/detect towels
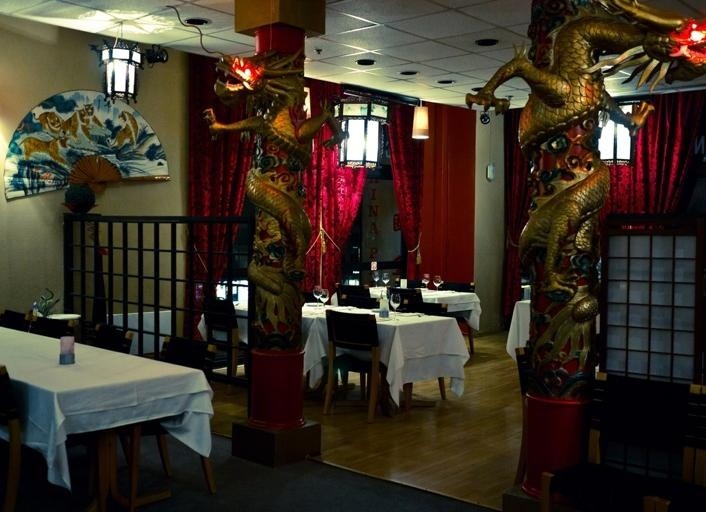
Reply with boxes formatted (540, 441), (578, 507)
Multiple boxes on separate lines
(335, 306), (357, 311)
(396, 312), (424, 317)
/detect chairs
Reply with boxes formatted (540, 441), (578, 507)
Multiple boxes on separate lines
(111, 337), (215, 512)
(0, 366), (95, 512)
(439, 281), (475, 355)
(387, 289), (447, 398)
(81, 323), (129, 353)
(0, 309), (25, 331)
(387, 288), (424, 313)
(336, 286), (372, 398)
(323, 311), (410, 421)
(203, 298), (246, 382)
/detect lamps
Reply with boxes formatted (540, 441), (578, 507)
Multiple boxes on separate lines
(413, 99), (430, 139)
(92, 36), (141, 102)
(599, 103), (639, 166)
(333, 89), (388, 168)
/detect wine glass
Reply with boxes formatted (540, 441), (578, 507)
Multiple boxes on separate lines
(381, 273), (390, 288)
(373, 270), (379, 289)
(433, 275), (442, 291)
(390, 294), (401, 320)
(421, 273), (431, 292)
(313, 285), (322, 307)
(321, 289), (330, 308)
